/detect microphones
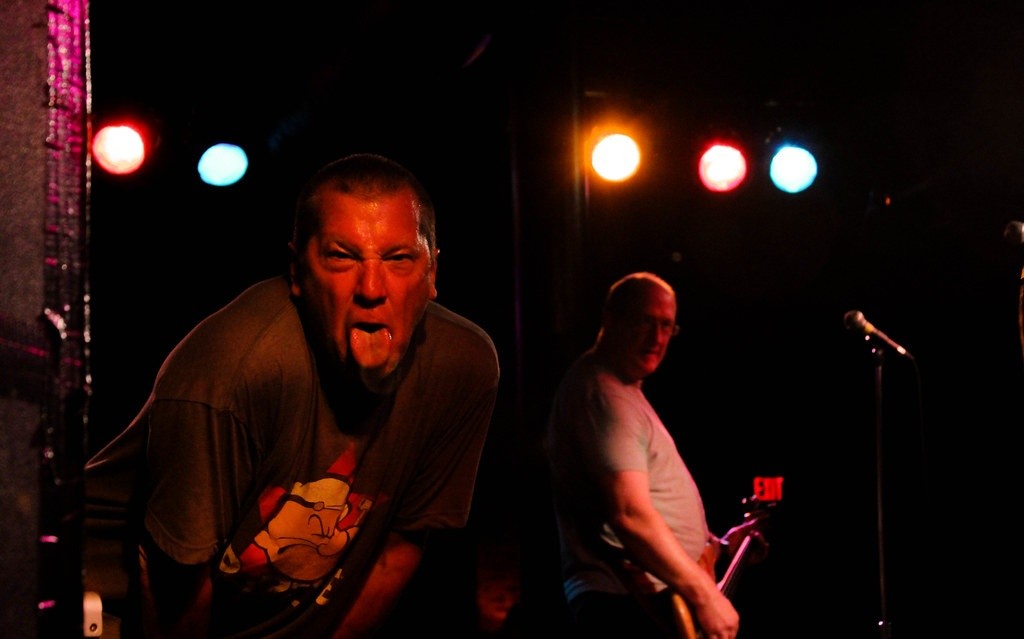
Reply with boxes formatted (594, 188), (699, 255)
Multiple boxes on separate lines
(845, 310), (914, 360)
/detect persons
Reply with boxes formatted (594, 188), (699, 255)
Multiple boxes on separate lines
(60, 153), (501, 639)
(542, 273), (772, 639)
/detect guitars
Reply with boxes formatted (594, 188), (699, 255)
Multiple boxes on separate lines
(666, 478), (783, 639)
